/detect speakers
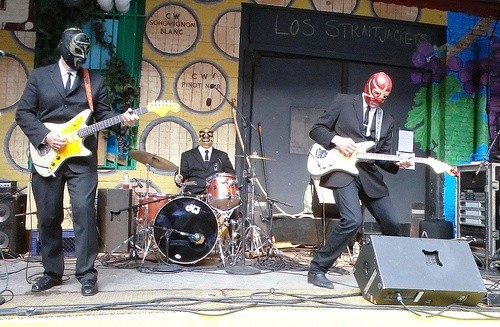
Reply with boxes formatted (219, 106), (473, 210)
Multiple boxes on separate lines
(353, 234), (486, 307)
(312, 178), (365, 218)
(0, 192), (27, 257)
(98, 189), (132, 254)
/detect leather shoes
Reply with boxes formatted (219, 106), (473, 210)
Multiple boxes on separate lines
(31, 275), (62, 292)
(81, 280), (98, 295)
(308, 270), (335, 289)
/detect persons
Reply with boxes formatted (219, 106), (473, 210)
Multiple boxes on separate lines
(174, 127), (242, 263)
(308, 72), (410, 289)
(15, 27), (139, 296)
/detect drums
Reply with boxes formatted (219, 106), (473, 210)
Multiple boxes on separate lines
(206, 173), (241, 210)
(152, 197), (218, 264)
(137, 192), (171, 225)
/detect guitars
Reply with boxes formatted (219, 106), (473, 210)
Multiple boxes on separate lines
(30, 99), (181, 177)
(307, 141), (457, 177)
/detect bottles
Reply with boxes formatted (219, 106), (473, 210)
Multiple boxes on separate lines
(352, 242), (359, 260)
(122, 171), (130, 189)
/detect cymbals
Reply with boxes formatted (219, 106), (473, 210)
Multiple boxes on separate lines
(231, 154), (272, 161)
(129, 150), (179, 173)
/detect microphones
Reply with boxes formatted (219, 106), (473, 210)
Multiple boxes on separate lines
(206, 77), (214, 106)
(175, 230), (201, 241)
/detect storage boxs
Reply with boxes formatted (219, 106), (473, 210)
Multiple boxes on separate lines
(32, 229), (76, 257)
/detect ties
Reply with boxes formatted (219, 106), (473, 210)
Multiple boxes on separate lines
(205, 150), (209, 167)
(65, 72), (72, 95)
(362, 105), (370, 136)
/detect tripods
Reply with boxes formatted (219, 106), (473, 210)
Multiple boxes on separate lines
(233, 158), (283, 264)
(96, 163), (179, 270)
